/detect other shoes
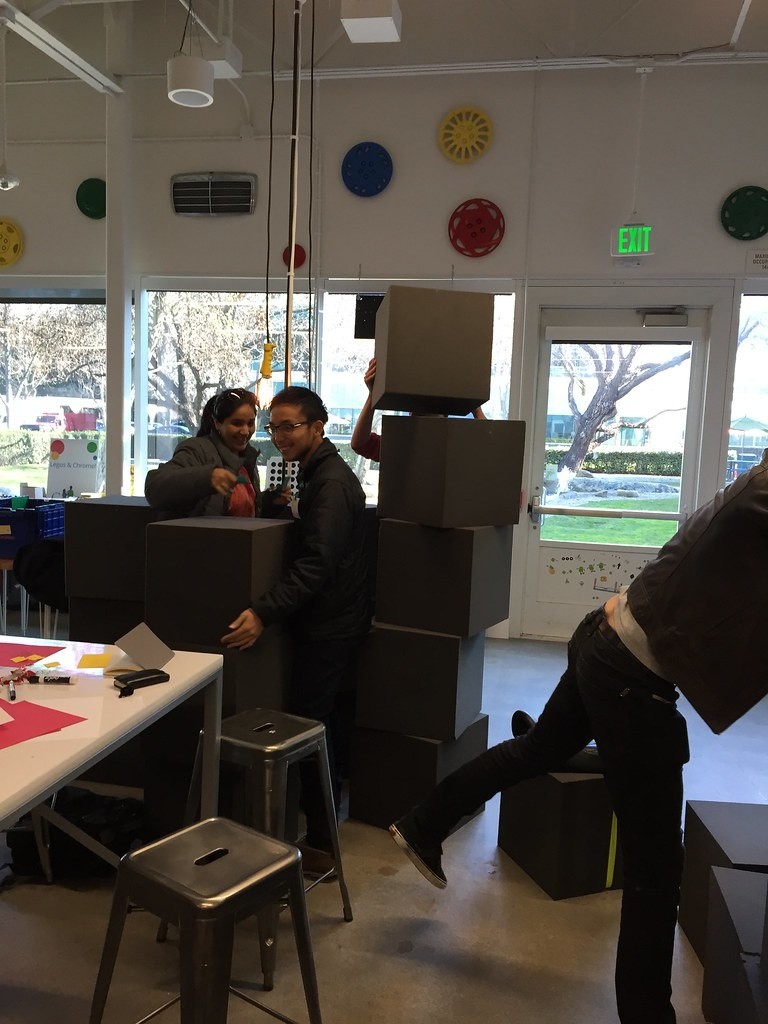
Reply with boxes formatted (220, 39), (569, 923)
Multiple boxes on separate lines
(290, 834), (339, 878)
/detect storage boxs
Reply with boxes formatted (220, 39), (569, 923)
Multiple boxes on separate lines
(373, 518), (513, 637)
(68, 598), (144, 645)
(353, 621), (486, 742)
(20, 487), (43, 499)
(678, 798), (768, 966)
(362, 503), (386, 614)
(739, 952), (768, 1024)
(0, 496), (64, 560)
(56, 738), (201, 842)
(143, 515), (296, 647)
(370, 285), (495, 417)
(346, 712), (489, 839)
(702, 865), (768, 1024)
(63, 494), (169, 602)
(141, 639), (299, 768)
(498, 767), (625, 901)
(378, 413), (527, 529)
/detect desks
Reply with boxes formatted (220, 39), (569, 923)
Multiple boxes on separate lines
(0, 633), (225, 884)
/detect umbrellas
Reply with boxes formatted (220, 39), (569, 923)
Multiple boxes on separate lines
(730, 416), (768, 459)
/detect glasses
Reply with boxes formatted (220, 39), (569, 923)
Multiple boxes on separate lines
(228, 388), (246, 401)
(264, 421), (311, 436)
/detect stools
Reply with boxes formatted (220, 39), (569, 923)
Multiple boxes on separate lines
(154, 705), (354, 993)
(87, 814), (325, 1024)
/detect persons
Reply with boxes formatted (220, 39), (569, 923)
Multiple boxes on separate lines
(144, 388), (292, 519)
(387, 453), (768, 1024)
(220, 384), (374, 884)
(351, 359), (488, 461)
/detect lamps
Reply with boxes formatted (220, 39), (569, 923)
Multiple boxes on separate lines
(166, 55), (216, 109)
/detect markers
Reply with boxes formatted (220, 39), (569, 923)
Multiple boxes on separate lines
(27, 676), (71, 685)
(9, 680), (16, 701)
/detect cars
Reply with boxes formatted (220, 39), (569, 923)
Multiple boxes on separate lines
(148, 425), (190, 434)
(20, 413), (65, 431)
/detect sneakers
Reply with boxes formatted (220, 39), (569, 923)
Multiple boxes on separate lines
(387, 804), (446, 889)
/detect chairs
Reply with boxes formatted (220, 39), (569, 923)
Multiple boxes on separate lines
(13, 536), (70, 638)
